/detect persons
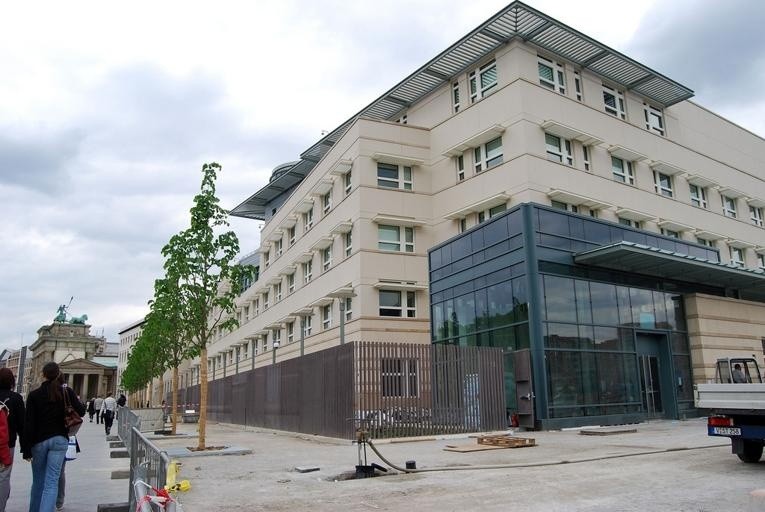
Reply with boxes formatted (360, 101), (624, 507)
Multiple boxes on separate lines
(114, 393), (127, 421)
(86, 397), (95, 422)
(76, 394), (84, 406)
(730, 363), (748, 383)
(52, 369), (66, 511)
(0, 366), (27, 511)
(20, 360), (85, 512)
(98, 392), (117, 435)
(92, 395), (104, 424)
(58, 304), (68, 314)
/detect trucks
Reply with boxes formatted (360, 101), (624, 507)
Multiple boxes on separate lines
(693, 383), (765, 463)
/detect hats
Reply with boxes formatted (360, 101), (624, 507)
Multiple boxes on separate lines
(42, 361), (59, 377)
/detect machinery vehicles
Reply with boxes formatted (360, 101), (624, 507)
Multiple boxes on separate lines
(714, 356), (763, 382)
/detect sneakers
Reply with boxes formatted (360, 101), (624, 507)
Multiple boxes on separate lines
(107, 427), (111, 434)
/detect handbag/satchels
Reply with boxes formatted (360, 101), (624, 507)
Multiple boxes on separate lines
(61, 385), (84, 437)
(64, 435), (77, 461)
